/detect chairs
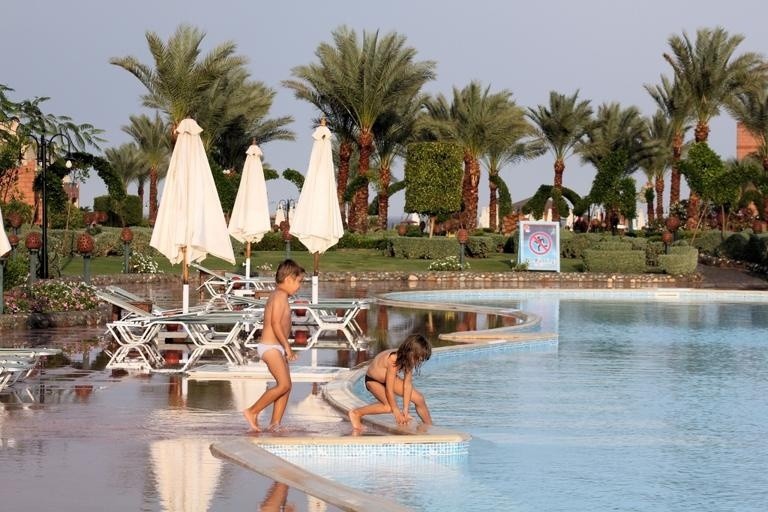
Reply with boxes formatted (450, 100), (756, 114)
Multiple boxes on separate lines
(1, 344), (64, 404)
(95, 262), (370, 376)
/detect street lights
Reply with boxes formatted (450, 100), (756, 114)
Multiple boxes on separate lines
(279, 198), (295, 259)
(18, 133), (73, 279)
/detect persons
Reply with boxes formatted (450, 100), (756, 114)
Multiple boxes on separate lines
(346, 331), (434, 432)
(257, 479), (294, 511)
(241, 258), (308, 432)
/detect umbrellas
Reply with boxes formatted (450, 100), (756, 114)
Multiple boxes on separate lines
(290, 343), (345, 512)
(287, 119), (347, 343)
(0, 208), (12, 261)
(229, 379), (269, 416)
(148, 115), (236, 360)
(227, 136), (272, 333)
(152, 362), (226, 511)
